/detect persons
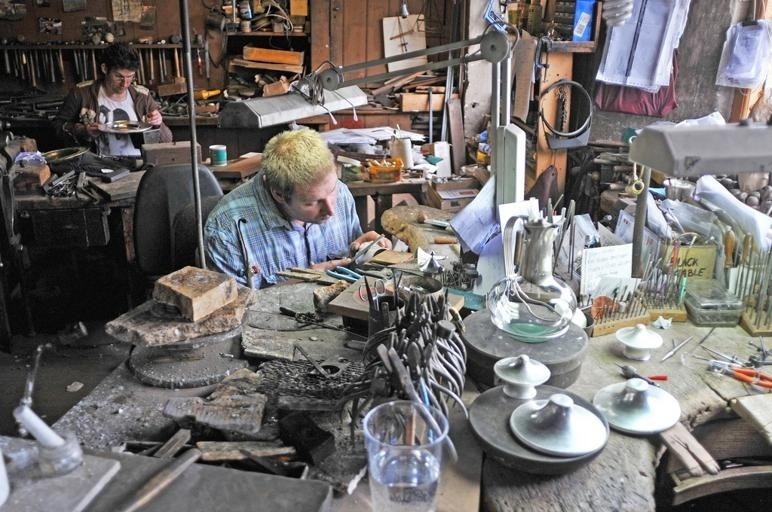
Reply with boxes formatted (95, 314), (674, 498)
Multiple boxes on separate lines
(194, 129), (393, 292)
(52, 42), (173, 160)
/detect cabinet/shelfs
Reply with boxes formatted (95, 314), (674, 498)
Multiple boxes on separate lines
(326, 107), (415, 131)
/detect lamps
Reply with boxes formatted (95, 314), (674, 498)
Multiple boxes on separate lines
(626, 119), (771, 179)
(216, 20), (527, 223)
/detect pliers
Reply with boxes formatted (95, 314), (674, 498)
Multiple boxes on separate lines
(326, 265), (362, 281)
(708, 359), (772, 388)
(376, 290), (468, 464)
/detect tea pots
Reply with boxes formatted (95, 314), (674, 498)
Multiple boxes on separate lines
(487, 213), (576, 344)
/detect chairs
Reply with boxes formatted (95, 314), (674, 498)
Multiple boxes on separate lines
(133, 163), (223, 276)
(175, 195), (224, 268)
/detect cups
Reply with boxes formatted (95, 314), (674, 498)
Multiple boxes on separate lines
(209, 144), (228, 167)
(364, 400), (450, 512)
